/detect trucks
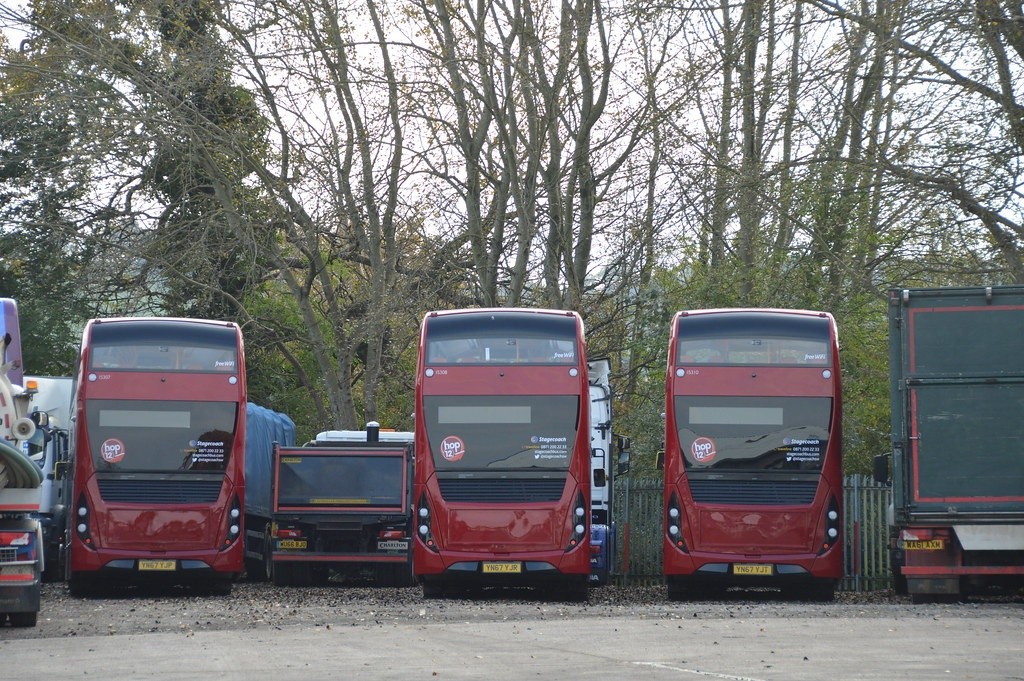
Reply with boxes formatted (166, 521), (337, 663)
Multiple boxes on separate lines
(1, 298), (73, 628)
(873, 284), (1024, 606)
(265, 421), (414, 588)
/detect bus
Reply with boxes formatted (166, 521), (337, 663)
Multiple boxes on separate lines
(657, 308), (844, 603)
(587, 352), (632, 589)
(66, 316), (247, 599)
(416, 307), (594, 605)
